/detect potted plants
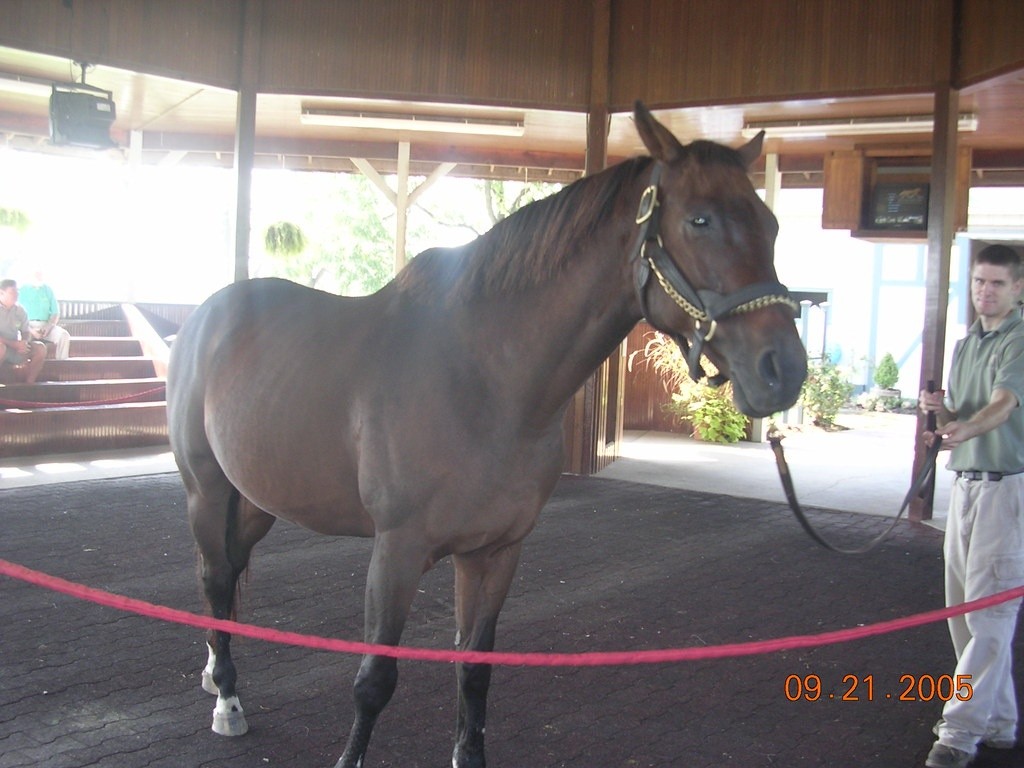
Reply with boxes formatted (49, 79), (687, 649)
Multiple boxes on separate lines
(870, 353), (900, 408)
(628, 329), (750, 445)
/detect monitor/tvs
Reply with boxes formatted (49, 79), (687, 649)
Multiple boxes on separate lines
(861, 157), (927, 231)
(48, 91), (116, 150)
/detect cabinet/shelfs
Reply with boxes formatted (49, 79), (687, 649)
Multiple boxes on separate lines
(822, 143), (974, 243)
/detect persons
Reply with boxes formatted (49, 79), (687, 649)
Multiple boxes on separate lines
(17, 265), (70, 360)
(919, 244), (1024, 768)
(0, 279), (47, 383)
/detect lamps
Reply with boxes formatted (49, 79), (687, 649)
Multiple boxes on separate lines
(742, 113), (979, 132)
(301, 107), (525, 137)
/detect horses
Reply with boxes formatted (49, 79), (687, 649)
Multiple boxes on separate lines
(165, 92), (810, 767)
